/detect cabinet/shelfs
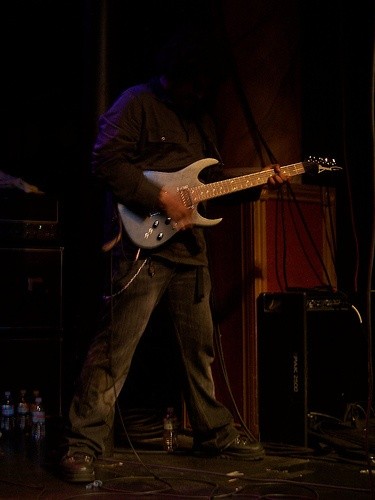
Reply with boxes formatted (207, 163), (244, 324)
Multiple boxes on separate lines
(1, 244), (65, 419)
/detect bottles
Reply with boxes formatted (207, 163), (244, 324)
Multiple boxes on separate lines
(30, 398), (45, 441)
(163, 408), (178, 453)
(0, 390), (40, 432)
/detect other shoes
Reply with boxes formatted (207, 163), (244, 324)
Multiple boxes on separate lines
(61, 452), (96, 482)
(192, 434), (265, 461)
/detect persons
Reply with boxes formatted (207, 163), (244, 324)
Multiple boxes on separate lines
(58, 37), (289, 483)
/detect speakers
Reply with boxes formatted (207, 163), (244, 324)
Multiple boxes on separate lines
(256, 287), (369, 455)
(0, 246), (64, 333)
(0, 334), (64, 420)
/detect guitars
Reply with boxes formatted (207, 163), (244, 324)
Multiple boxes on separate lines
(115, 156), (344, 251)
